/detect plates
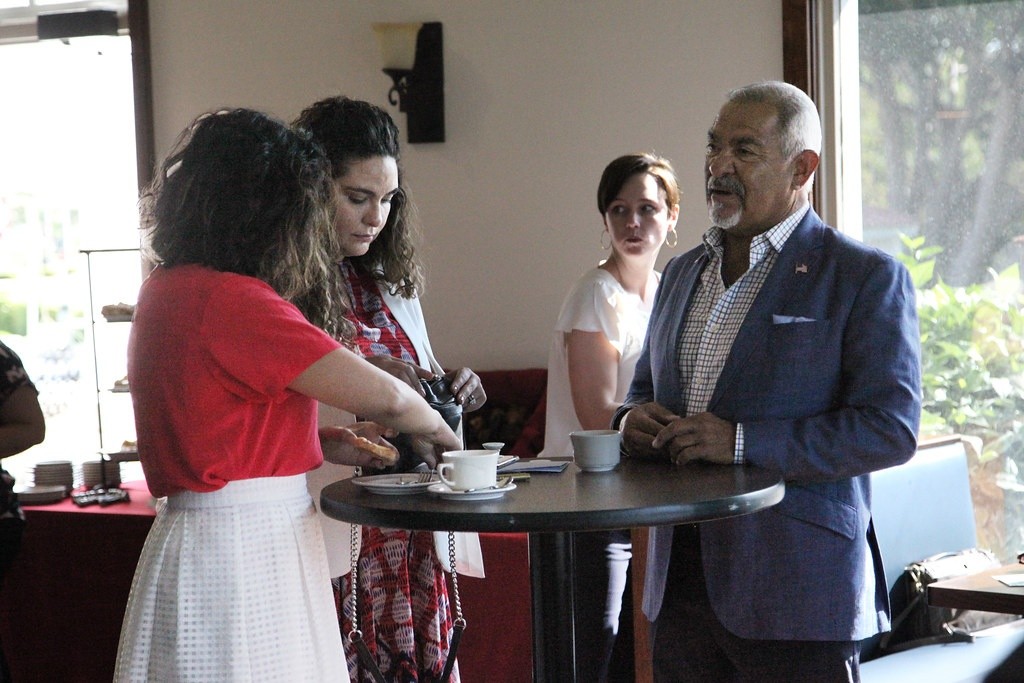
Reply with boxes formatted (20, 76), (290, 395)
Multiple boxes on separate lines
(427, 482), (517, 500)
(351, 473), (440, 494)
(16, 485), (65, 503)
(81, 461), (121, 489)
(30, 461), (74, 495)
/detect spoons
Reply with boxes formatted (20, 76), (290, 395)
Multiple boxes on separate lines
(464, 475), (513, 493)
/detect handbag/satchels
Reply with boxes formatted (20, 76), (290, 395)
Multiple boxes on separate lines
(359, 370), (463, 475)
(878, 548), (1021, 658)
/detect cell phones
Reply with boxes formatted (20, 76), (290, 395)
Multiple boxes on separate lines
(497, 455), (519, 469)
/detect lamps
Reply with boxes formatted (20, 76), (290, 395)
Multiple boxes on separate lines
(371, 21), (444, 144)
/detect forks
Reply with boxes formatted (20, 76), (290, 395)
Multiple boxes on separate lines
(418, 470), (433, 482)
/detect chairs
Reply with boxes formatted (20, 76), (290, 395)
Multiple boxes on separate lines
(860, 436), (1024, 683)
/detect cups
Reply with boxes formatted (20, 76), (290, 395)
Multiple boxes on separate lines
(438, 450), (500, 492)
(569, 430), (621, 472)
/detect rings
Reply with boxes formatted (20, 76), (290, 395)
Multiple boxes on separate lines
(468, 395), (476, 405)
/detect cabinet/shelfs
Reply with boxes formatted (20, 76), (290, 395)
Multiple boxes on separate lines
(80, 248), (140, 491)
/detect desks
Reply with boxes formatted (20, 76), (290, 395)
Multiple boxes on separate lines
(319, 456), (787, 683)
(10, 479), (157, 682)
(927, 560), (1024, 615)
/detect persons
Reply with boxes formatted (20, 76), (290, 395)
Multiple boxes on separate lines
(112, 104), (466, 683)
(527, 151), (682, 682)
(0, 343), (47, 681)
(616, 77), (920, 682)
(285, 93), (491, 683)
